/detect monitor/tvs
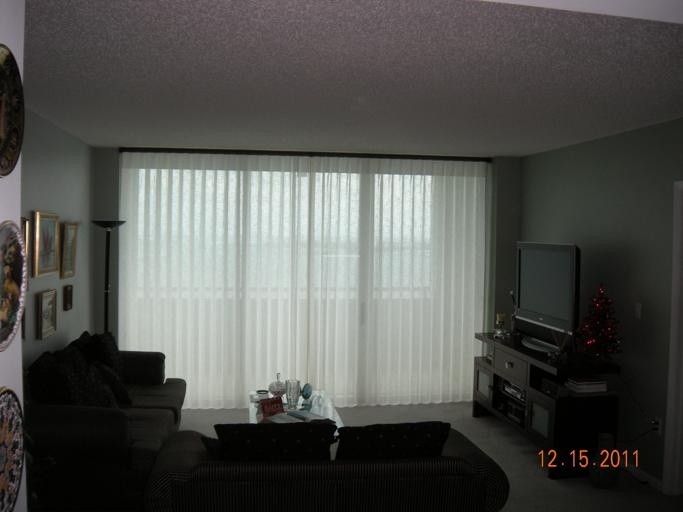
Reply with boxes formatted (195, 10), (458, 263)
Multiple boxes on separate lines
(514, 241), (580, 352)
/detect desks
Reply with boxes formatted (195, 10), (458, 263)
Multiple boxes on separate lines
(246, 391), (345, 436)
(472, 323), (621, 454)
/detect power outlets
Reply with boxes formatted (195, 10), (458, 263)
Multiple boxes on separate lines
(650, 418), (664, 436)
(633, 302), (644, 321)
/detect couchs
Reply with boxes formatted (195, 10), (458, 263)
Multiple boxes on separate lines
(149, 429), (510, 512)
(19, 349), (186, 478)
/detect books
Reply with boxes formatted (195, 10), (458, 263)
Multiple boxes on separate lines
(264, 410), (326, 423)
(564, 377), (607, 394)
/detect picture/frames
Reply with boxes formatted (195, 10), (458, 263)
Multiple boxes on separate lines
(38, 288), (58, 342)
(31, 208), (60, 280)
(64, 284), (73, 311)
(61, 221), (78, 278)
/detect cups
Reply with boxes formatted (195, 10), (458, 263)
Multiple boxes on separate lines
(256, 390), (269, 401)
(286, 380), (302, 410)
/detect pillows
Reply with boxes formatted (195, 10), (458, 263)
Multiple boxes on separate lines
(75, 328), (130, 388)
(35, 347), (117, 414)
(338, 419), (450, 459)
(214, 419), (335, 465)
